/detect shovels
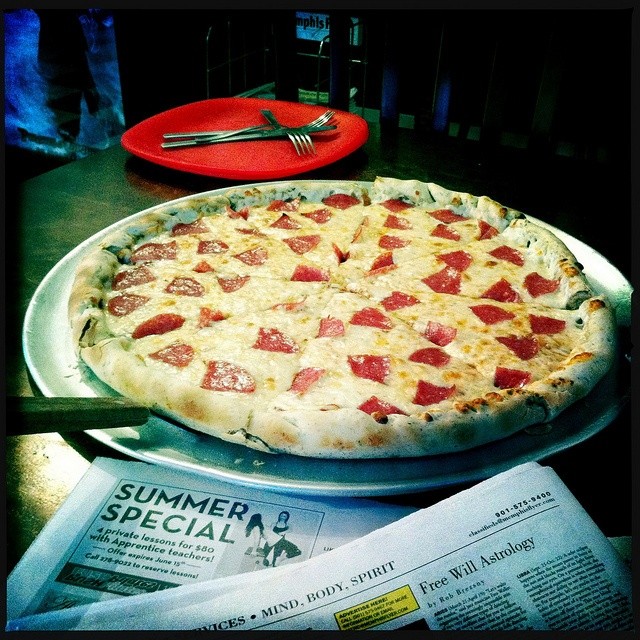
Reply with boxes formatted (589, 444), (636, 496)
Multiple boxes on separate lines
(5, 395), (209, 438)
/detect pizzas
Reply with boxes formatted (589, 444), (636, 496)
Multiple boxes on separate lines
(66, 175), (615, 462)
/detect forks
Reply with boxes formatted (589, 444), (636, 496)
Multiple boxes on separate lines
(163, 112), (342, 151)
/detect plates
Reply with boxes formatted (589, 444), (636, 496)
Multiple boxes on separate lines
(22, 180), (633, 496)
(119, 94), (371, 179)
